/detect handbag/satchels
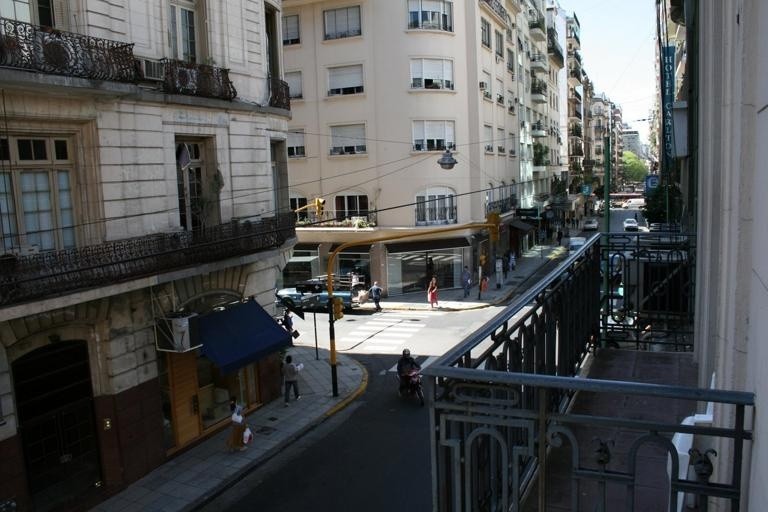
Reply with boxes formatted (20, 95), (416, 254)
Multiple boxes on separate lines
(231, 411), (243, 424)
(242, 427), (254, 445)
(283, 320), (289, 327)
(292, 329), (300, 339)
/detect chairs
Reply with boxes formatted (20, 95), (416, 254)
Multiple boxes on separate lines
(32, 31), (84, 76)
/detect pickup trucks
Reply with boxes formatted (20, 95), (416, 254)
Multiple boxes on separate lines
(274, 273), (366, 311)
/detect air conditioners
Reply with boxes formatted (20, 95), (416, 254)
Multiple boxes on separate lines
(172, 66), (198, 91)
(521, 121), (529, 128)
(133, 58), (166, 83)
(479, 80), (487, 91)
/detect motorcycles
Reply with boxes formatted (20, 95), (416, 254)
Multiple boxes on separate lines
(401, 367), (424, 405)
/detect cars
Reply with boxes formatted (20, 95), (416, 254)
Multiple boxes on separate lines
(583, 218), (599, 231)
(568, 236), (586, 257)
(624, 217), (638, 231)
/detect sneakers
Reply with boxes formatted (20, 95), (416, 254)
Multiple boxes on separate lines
(296, 395), (302, 401)
(239, 446), (248, 452)
(284, 402), (290, 407)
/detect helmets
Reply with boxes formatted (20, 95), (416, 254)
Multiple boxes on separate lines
(403, 348), (410, 358)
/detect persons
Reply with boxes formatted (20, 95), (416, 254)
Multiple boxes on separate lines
(482, 209), (581, 296)
(280, 356), (302, 407)
(461, 265), (470, 297)
(367, 281), (383, 312)
(396, 348), (420, 396)
(283, 308), (294, 347)
(228, 405), (248, 452)
(427, 274), (438, 308)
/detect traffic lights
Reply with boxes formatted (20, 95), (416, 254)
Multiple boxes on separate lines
(315, 198), (325, 217)
(334, 296), (344, 320)
(480, 254), (486, 268)
